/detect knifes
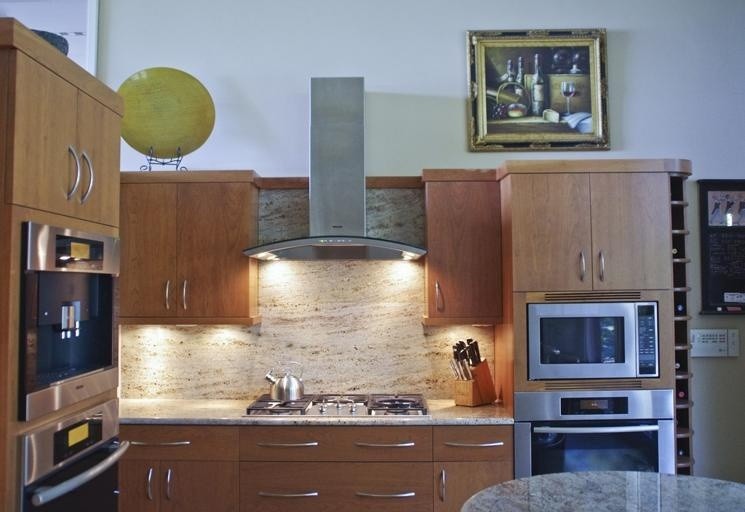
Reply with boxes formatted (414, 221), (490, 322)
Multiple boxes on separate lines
(447, 336), (498, 408)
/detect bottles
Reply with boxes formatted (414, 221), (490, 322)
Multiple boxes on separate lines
(505, 59), (516, 93)
(515, 56), (526, 96)
(530, 54), (550, 116)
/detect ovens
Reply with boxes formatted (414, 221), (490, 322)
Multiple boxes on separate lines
(20, 228), (123, 420)
(16, 397), (126, 511)
(515, 394), (675, 475)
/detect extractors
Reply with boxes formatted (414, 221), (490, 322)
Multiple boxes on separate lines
(242, 75), (427, 265)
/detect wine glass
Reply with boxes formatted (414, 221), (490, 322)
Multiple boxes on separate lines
(561, 81), (577, 117)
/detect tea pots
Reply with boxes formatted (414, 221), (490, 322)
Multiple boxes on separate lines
(265, 359), (307, 401)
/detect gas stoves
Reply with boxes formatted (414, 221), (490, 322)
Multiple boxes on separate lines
(247, 390), (429, 417)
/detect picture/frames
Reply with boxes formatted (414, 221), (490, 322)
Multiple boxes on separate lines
(462, 26), (613, 153)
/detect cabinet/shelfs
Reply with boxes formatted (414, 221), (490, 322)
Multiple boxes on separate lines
(434, 425), (515, 511)
(0, 15), (124, 226)
(120, 424), (240, 512)
(490, 156), (692, 293)
(414, 163), (504, 326)
(241, 424), (433, 512)
(117, 169), (261, 326)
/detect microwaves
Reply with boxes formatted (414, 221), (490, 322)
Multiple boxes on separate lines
(528, 302), (659, 380)
